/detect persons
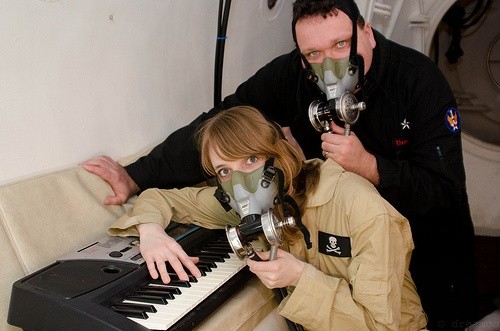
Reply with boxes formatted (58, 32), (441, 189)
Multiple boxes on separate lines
(80, 0), (480, 331)
(124, 105), (430, 331)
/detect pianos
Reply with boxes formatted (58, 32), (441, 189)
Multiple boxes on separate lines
(7, 217), (259, 330)
